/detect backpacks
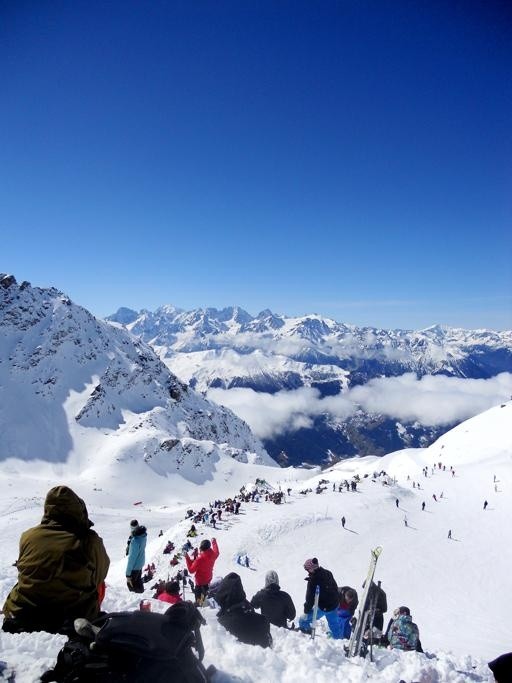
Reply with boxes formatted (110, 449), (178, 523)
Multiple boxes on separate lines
(40, 599), (216, 683)
(216, 603), (271, 648)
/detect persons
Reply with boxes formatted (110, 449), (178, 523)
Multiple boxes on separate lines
(251, 569), (298, 628)
(335, 584), (360, 639)
(362, 578), (388, 644)
(448, 530), (451, 538)
(286, 469), (388, 496)
(341, 517), (345, 527)
(395, 462), (454, 526)
(300, 556), (344, 639)
(383, 604), (424, 653)
(210, 478), (283, 514)
(483, 500), (488, 509)
(146, 508), (223, 603)
(213, 570), (276, 651)
(238, 555), (249, 567)
(124, 518), (150, 592)
(2, 483), (111, 636)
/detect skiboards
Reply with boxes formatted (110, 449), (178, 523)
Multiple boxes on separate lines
(310, 586), (320, 640)
(343, 546), (382, 660)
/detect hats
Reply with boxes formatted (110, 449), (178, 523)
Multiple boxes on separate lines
(131, 520), (139, 529)
(304, 558), (318, 571)
(265, 570), (279, 586)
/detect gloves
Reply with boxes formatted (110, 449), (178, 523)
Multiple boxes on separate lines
(127, 577), (133, 588)
